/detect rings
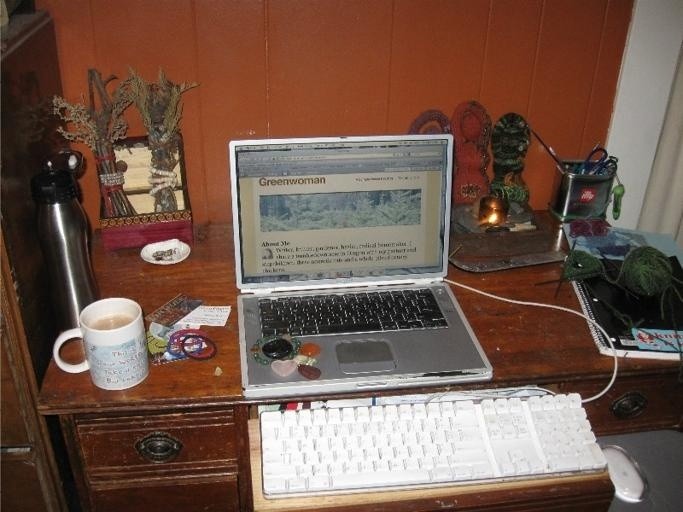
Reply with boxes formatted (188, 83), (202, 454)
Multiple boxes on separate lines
(613, 184), (625, 220)
(573, 141), (618, 175)
(532, 129), (567, 172)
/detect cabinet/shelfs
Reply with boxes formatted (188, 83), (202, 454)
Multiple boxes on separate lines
(31, 168), (98, 329)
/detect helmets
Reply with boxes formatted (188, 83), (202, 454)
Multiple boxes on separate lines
(141, 238), (190, 265)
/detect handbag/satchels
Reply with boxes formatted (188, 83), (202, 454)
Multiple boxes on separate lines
(250, 331), (301, 366)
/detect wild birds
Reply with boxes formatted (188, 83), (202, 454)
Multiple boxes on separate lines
(228, 134), (494, 399)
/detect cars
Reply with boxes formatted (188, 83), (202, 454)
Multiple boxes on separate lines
(53, 297), (148, 391)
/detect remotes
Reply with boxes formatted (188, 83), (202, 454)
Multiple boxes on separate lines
(602, 444), (650, 505)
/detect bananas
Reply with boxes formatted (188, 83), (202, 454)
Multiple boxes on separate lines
(258, 392), (608, 499)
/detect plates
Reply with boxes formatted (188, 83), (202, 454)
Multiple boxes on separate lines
(0, 12), (61, 512)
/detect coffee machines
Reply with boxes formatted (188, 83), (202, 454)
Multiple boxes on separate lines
(584, 147), (617, 175)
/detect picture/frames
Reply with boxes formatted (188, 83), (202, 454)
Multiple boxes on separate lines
(35, 210), (683, 512)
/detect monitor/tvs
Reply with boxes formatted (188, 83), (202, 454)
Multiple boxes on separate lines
(563, 253), (683, 360)
